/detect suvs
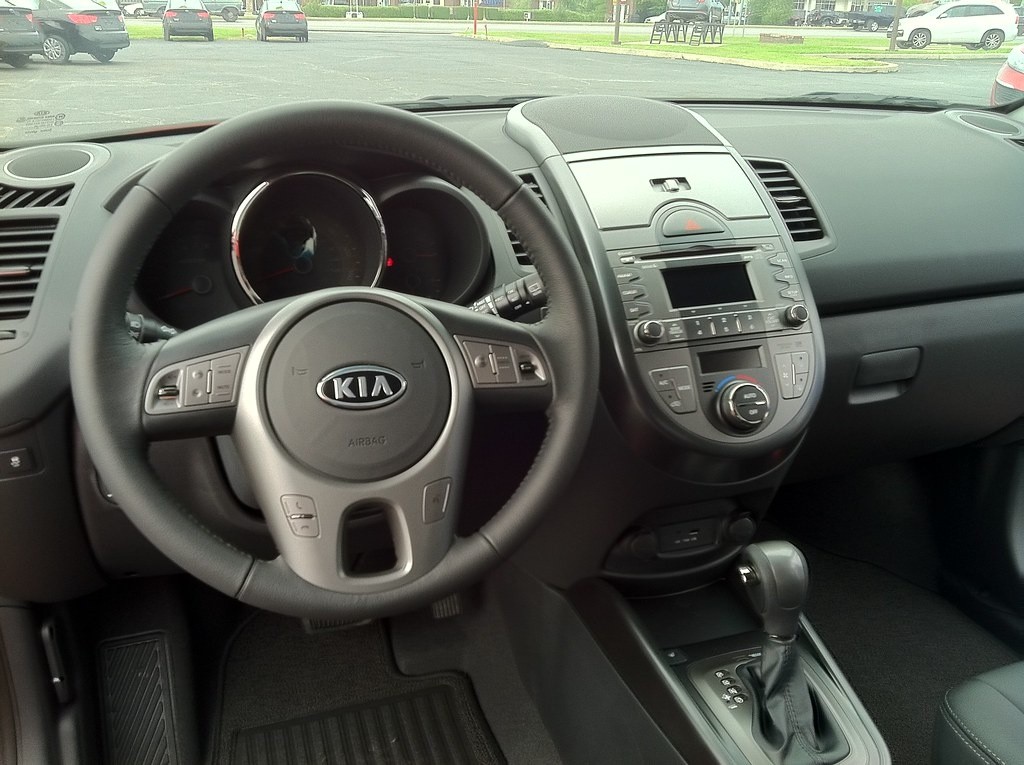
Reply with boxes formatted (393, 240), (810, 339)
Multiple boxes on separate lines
(123, 0), (246, 23)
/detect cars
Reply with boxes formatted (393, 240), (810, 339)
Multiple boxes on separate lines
(805, 0), (1024, 50)
(162, 0), (213, 41)
(642, 0), (748, 25)
(255, 0), (309, 43)
(29, 0), (132, 65)
(0, 6), (45, 69)
